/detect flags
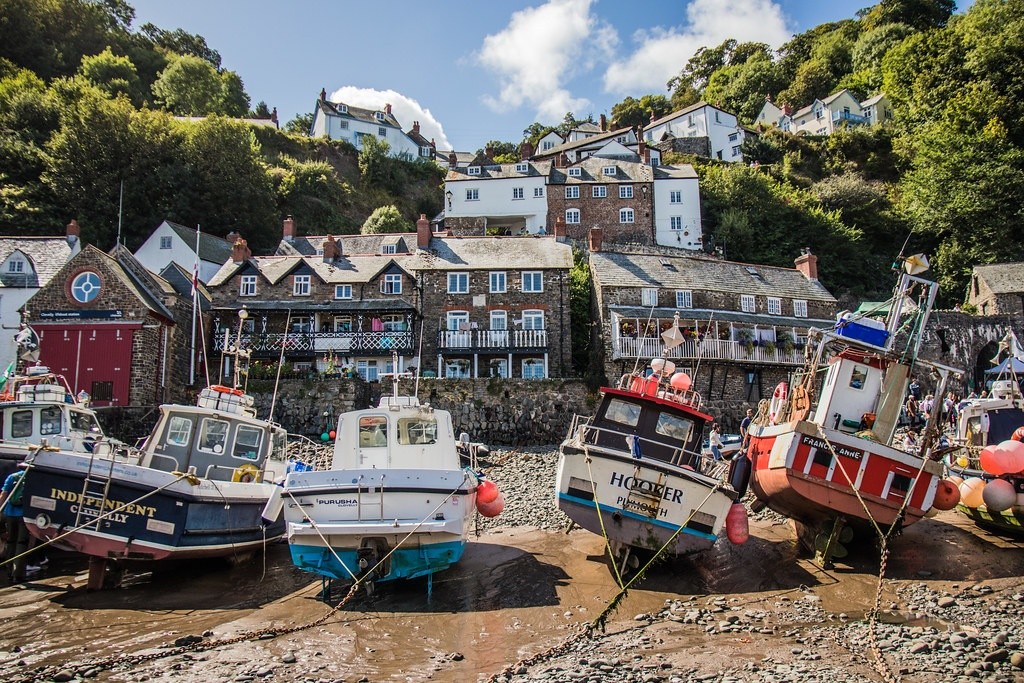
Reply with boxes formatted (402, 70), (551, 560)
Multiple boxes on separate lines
(192, 262), (198, 291)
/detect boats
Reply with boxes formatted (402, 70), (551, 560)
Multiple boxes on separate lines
(945, 348), (1024, 525)
(19, 309), (297, 594)
(279, 344), (479, 605)
(555, 309), (740, 581)
(0, 321), (141, 544)
(703, 433), (744, 458)
(744, 269), (967, 568)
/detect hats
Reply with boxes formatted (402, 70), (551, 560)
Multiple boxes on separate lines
(908, 395), (915, 399)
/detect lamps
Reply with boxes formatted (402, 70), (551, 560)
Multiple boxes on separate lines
(744, 366), (756, 384)
(641, 185), (649, 199)
(735, 124), (741, 137)
(446, 190), (453, 212)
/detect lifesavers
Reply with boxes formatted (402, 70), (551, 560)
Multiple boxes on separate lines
(210, 384), (243, 396)
(769, 382), (787, 424)
(1010, 427), (1024, 475)
(234, 464), (262, 483)
(658, 391), (698, 407)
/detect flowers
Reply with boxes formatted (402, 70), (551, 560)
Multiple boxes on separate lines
(621, 319), (731, 341)
(247, 357), (279, 373)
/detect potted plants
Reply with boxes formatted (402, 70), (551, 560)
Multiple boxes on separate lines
(737, 328), (808, 355)
(242, 344), (358, 380)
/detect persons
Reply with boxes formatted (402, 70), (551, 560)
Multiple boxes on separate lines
(709, 422), (725, 461)
(740, 408), (757, 448)
(936, 430), (949, 450)
(0, 462), (30, 584)
(904, 430), (921, 456)
(907, 390), (987, 430)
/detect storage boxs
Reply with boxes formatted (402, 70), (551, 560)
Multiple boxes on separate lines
(196, 387), (247, 417)
(19, 384), (66, 404)
(836, 314), (890, 347)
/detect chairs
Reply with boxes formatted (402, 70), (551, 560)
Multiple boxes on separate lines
(360, 432), (371, 446)
